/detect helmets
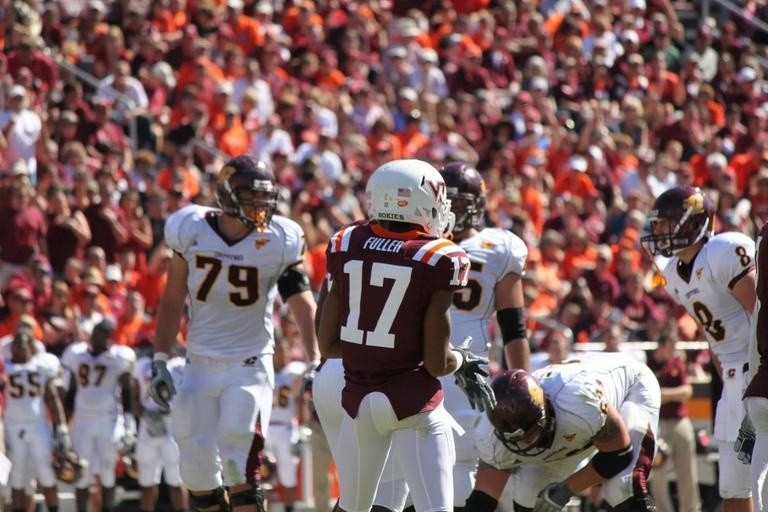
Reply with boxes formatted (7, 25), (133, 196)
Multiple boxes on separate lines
(365, 160), (454, 236)
(212, 154), (279, 230)
(641, 186), (716, 256)
(485, 371), (555, 457)
(52, 446), (87, 483)
(439, 162), (486, 231)
(121, 450), (138, 481)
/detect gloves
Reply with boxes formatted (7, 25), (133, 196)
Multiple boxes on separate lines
(455, 369), (499, 412)
(454, 337), (489, 387)
(121, 433), (138, 456)
(147, 359), (176, 410)
(534, 483), (571, 512)
(298, 359), (323, 397)
(735, 415), (755, 465)
(54, 423), (73, 451)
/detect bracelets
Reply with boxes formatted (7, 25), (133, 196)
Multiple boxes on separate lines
(451, 351), (463, 376)
(152, 351), (170, 362)
(306, 359), (321, 370)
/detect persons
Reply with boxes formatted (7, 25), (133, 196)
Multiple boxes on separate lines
(438, 162), (532, 511)
(129, 336), (191, 510)
(153, 155), (321, 511)
(54, 318), (130, 511)
(468, 355), (663, 511)
(650, 330), (706, 511)
(640, 188), (763, 511)
(750, 213), (766, 512)
(316, 163), (465, 512)
(0, 1), (768, 372)
(0, 332), (76, 512)
